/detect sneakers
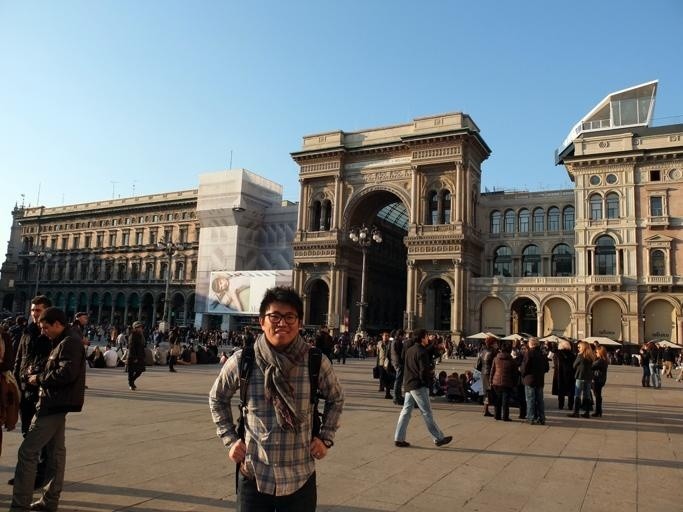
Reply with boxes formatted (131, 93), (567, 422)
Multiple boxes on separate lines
(581, 413), (589, 418)
(567, 413), (579, 418)
(436, 437), (452, 446)
(385, 395), (393, 399)
(395, 441), (409, 447)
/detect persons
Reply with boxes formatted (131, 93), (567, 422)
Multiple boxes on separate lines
(662, 345), (682, 381)
(143, 326), (262, 373)
(299, 325), (348, 366)
(377, 329), (419, 409)
(7, 294), (55, 489)
(207, 285), (344, 511)
(1, 316), (28, 373)
(71, 312), (131, 368)
(211, 276), (251, 311)
(607, 348), (640, 367)
(430, 335), (609, 424)
(124, 318), (149, 391)
(640, 343), (665, 389)
(9, 306), (87, 511)
(392, 327), (453, 451)
(350, 334), (376, 359)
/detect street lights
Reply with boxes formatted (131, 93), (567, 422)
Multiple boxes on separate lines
(20, 246), (56, 306)
(149, 230), (190, 346)
(338, 222), (389, 350)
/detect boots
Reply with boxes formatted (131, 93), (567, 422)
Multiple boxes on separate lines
(592, 398), (602, 417)
(483, 404), (494, 416)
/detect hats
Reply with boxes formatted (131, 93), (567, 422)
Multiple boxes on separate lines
(396, 328), (404, 336)
(390, 329), (396, 335)
(132, 321), (142, 328)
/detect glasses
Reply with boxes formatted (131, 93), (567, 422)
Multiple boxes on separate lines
(263, 313), (299, 324)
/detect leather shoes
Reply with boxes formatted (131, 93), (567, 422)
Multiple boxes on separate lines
(30, 502), (58, 512)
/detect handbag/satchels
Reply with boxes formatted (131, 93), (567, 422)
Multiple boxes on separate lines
(334, 344), (340, 354)
(661, 366), (667, 375)
(373, 366), (379, 378)
(474, 356), (482, 372)
(2, 370), (21, 431)
(513, 375), (521, 387)
(170, 345), (181, 356)
(383, 357), (389, 369)
